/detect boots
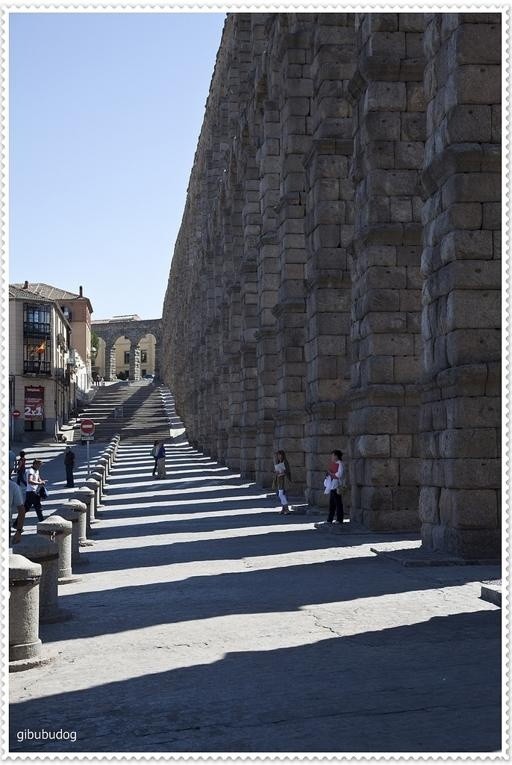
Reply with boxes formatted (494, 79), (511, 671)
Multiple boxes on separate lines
(281, 505), (291, 515)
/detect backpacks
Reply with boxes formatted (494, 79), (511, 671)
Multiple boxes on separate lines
(337, 462), (348, 495)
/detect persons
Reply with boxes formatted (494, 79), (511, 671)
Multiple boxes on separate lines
(63, 445), (75, 489)
(9, 444), (18, 480)
(13, 458), (50, 532)
(95, 373), (101, 386)
(9, 479), (25, 546)
(157, 443), (168, 479)
(271, 450), (293, 517)
(324, 448), (344, 524)
(16, 450), (28, 487)
(152, 440), (161, 478)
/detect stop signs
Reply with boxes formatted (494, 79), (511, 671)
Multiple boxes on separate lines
(12, 410), (20, 417)
(80, 419), (95, 436)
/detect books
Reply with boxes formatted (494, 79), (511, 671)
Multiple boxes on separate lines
(328, 462), (339, 480)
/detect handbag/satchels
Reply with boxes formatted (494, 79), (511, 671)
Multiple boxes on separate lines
(284, 475), (293, 491)
(39, 486), (48, 499)
